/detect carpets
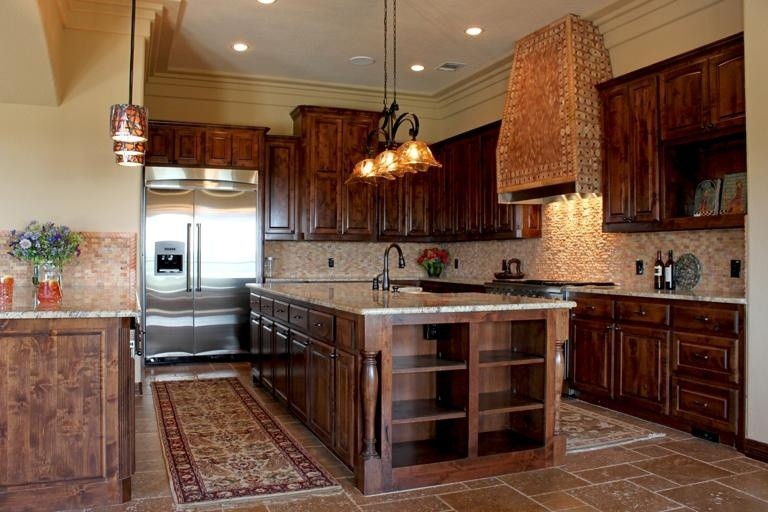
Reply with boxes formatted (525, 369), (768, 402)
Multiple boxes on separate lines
(149, 376), (343, 512)
(560, 401), (667, 454)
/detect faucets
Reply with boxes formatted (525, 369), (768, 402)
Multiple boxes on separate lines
(382, 242), (406, 290)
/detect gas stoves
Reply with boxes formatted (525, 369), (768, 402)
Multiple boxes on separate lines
(483, 278), (621, 300)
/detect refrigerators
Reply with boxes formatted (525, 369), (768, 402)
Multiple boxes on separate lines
(141, 165), (258, 365)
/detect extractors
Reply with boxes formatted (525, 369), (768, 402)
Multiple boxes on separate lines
(496, 181), (602, 205)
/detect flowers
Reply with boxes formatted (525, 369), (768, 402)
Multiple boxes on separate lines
(417, 247), (450, 275)
(6, 219), (84, 280)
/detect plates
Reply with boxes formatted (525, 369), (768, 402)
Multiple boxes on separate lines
(674, 254), (702, 291)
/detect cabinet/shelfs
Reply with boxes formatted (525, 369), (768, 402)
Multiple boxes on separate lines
(249, 292), (274, 393)
(569, 296), (670, 416)
(477, 319), (547, 457)
(658, 43), (745, 141)
(274, 299), (310, 426)
(308, 309), (355, 468)
(671, 304), (740, 436)
(391, 322), (470, 468)
(262, 104), (543, 243)
(147, 124), (259, 168)
(600, 75), (660, 225)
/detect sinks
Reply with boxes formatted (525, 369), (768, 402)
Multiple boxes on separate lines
(398, 286), (433, 294)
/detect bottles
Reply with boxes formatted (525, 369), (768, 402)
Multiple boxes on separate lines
(264, 257), (275, 278)
(653, 250), (664, 289)
(664, 249), (677, 291)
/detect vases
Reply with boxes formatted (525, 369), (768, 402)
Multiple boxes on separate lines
(427, 262), (442, 278)
(34, 263), (63, 312)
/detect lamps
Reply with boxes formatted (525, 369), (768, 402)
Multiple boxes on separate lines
(110, 0), (149, 167)
(343, 0), (443, 188)
